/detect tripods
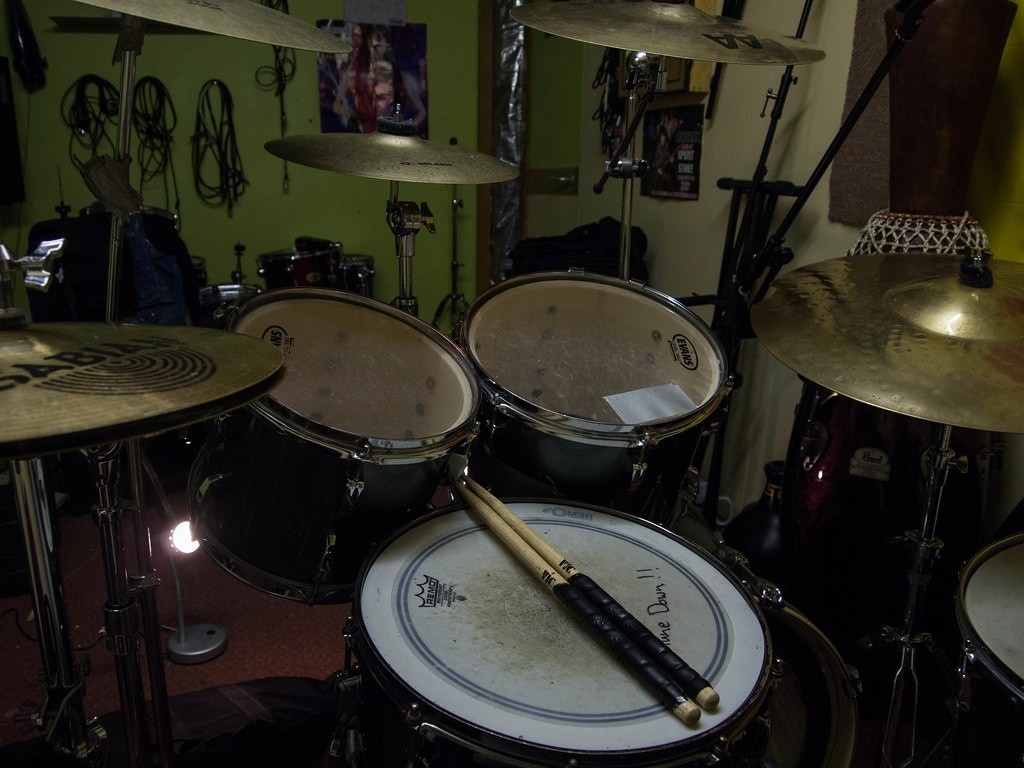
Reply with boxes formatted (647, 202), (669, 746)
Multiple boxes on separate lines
(841, 422), (968, 768)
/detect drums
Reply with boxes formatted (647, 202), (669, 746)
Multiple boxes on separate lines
(346, 493), (777, 768)
(183, 287), (483, 603)
(460, 268), (731, 531)
(778, 384), (994, 679)
(952, 532), (1024, 768)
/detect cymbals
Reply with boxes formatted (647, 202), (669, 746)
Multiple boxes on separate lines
(264, 132), (520, 185)
(751, 252), (1024, 434)
(73, 0), (354, 54)
(0, 320), (287, 463)
(508, 0), (827, 66)
